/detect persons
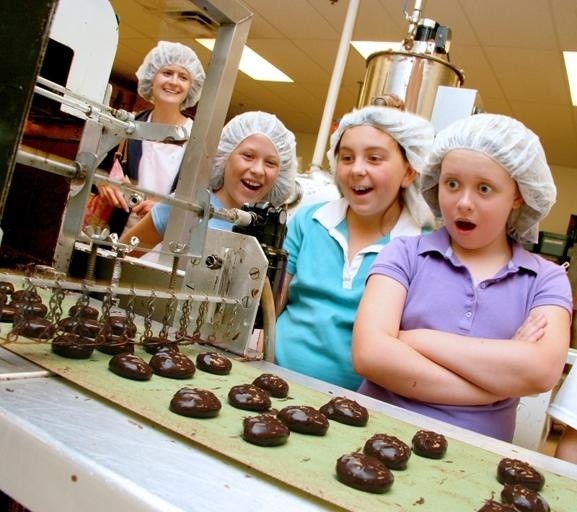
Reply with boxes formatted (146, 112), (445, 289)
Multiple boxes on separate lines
(118, 110), (298, 258)
(545, 358), (577, 467)
(90, 40), (207, 239)
(274, 105), (436, 391)
(352, 113), (574, 444)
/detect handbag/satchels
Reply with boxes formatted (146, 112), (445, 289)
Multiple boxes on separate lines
(81, 158), (131, 233)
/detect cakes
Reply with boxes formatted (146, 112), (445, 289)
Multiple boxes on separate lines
(336, 453), (395, 493)
(319, 396), (369, 426)
(143, 336), (179, 355)
(108, 316), (136, 338)
(0, 306), (23, 322)
(59, 317), (101, 338)
(277, 405), (329, 436)
(228, 384), (271, 411)
(14, 302), (48, 318)
(169, 387), (222, 418)
(497, 457), (545, 491)
(251, 374), (289, 398)
(502, 483), (551, 512)
(476, 500), (518, 512)
(108, 352), (153, 381)
(149, 349), (195, 379)
(243, 415), (290, 446)
(0, 293), (13, 306)
(15, 316), (52, 339)
(0, 282), (14, 294)
(196, 352), (232, 375)
(95, 335), (134, 355)
(412, 430), (448, 459)
(70, 305), (99, 320)
(13, 290), (42, 305)
(52, 335), (95, 359)
(364, 433), (411, 469)
